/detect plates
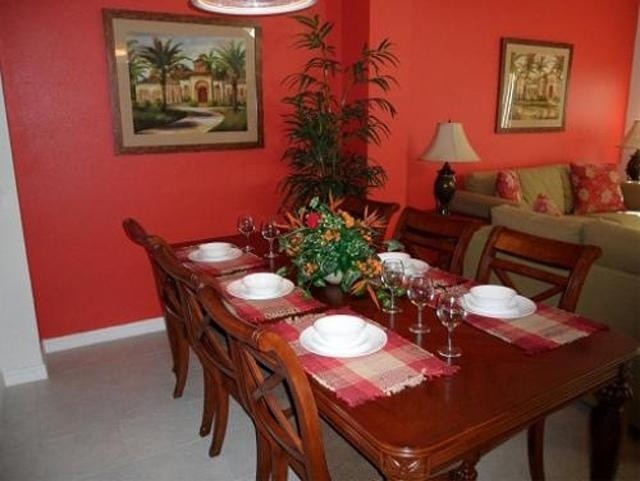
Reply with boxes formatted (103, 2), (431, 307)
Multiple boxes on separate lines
(459, 292), (539, 318)
(300, 324), (388, 358)
(226, 278), (295, 301)
(189, 248), (242, 262)
(403, 258), (431, 271)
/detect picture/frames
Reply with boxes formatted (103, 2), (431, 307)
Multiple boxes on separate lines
(100, 9), (265, 153)
(496, 34), (573, 134)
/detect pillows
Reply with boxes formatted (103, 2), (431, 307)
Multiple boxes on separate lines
(496, 166), (524, 205)
(535, 187), (560, 217)
(567, 161), (628, 215)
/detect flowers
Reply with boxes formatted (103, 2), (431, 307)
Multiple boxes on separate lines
(279, 194), (398, 311)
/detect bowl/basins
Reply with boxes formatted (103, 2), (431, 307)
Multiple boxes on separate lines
(377, 251), (413, 268)
(314, 312), (364, 345)
(242, 272), (284, 293)
(470, 284), (516, 309)
(198, 242), (231, 256)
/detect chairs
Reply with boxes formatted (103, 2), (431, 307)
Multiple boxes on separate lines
(475, 225), (601, 480)
(337, 193), (397, 249)
(154, 246), (296, 455)
(123, 220), (227, 395)
(196, 285), (469, 480)
(393, 205), (489, 278)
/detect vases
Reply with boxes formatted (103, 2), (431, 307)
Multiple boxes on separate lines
(320, 277), (350, 306)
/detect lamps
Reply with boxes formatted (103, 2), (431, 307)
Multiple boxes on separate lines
(187, 0), (319, 18)
(417, 119), (481, 216)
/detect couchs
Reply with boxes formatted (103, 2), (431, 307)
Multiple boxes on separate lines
(468, 157), (640, 410)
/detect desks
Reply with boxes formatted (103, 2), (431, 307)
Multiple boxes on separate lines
(162, 223), (640, 480)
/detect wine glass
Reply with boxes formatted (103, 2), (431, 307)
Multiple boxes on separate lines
(436, 290), (464, 358)
(237, 213), (256, 251)
(261, 216), (280, 257)
(406, 272), (434, 334)
(383, 257), (405, 313)
